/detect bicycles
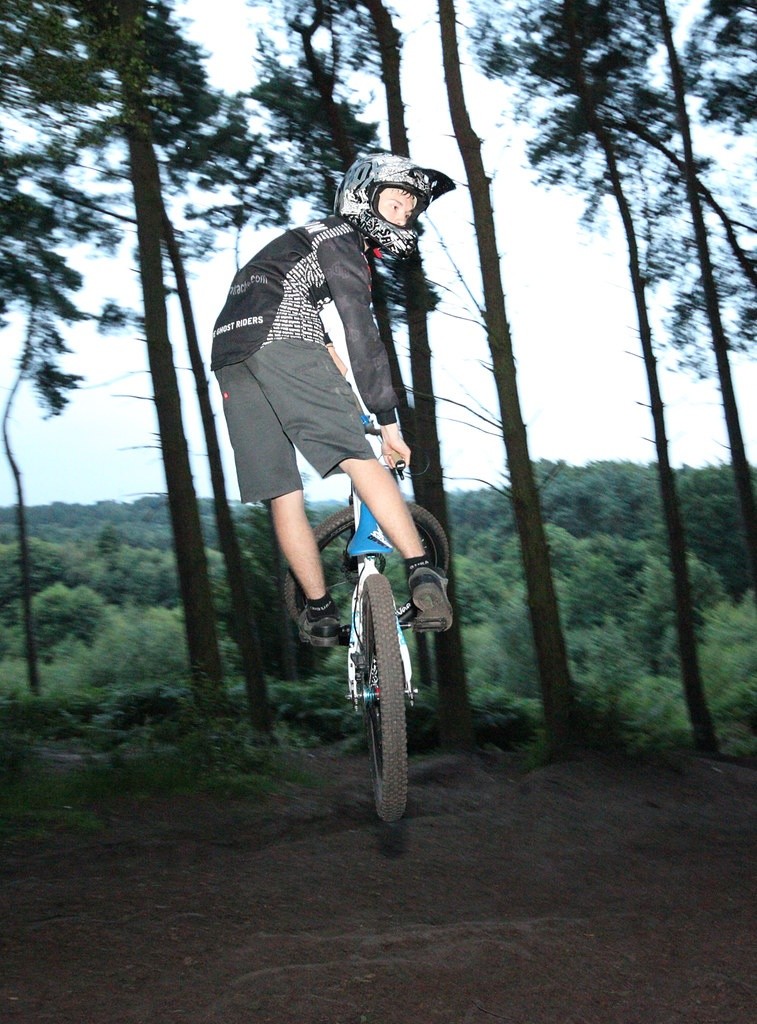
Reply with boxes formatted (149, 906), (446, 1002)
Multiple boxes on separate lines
(285, 382), (448, 821)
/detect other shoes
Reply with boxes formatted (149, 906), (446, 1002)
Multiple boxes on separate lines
(408, 565), (452, 631)
(297, 608), (340, 647)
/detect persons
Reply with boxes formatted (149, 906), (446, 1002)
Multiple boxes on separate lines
(211, 153), (453, 647)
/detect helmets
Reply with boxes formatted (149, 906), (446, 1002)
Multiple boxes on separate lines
(335, 149), (435, 262)
